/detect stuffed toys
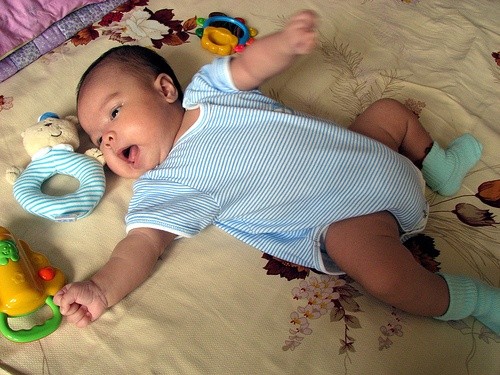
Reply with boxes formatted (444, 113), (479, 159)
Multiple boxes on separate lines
(5, 112), (107, 225)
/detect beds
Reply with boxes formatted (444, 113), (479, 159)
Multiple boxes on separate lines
(0, 0), (500, 375)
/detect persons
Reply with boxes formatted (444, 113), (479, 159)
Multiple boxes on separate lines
(53, 9), (500, 338)
